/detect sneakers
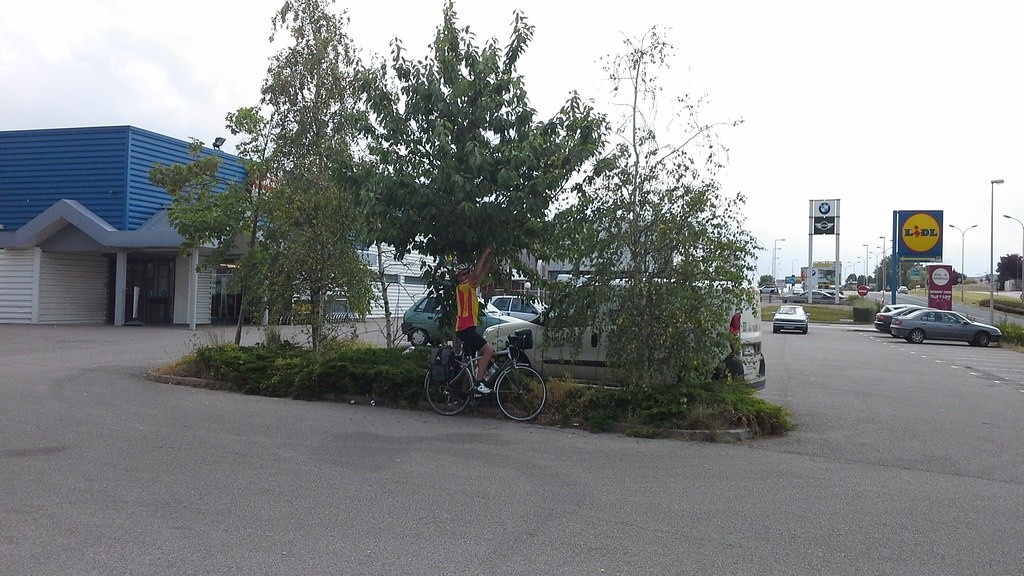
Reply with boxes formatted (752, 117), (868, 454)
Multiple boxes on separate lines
(473, 382), (493, 394)
(472, 391), (484, 397)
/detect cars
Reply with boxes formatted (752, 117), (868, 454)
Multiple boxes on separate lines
(401, 291), (553, 352)
(890, 309), (1002, 347)
(781, 287), (849, 305)
(771, 305), (811, 335)
(897, 285), (909, 294)
(758, 284), (778, 295)
(874, 304), (957, 340)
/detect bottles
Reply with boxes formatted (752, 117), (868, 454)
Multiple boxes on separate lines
(474, 364), (479, 380)
(483, 362), (501, 381)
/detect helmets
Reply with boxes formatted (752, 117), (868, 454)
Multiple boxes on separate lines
(454, 263), (471, 274)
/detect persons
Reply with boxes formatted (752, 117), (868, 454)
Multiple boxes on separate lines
(454, 241), (498, 398)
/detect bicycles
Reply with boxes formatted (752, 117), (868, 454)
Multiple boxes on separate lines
(424, 334), (548, 423)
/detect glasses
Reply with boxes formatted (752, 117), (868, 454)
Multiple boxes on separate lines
(458, 271), (472, 276)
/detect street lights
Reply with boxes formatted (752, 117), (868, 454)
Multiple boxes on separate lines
(775, 238), (785, 281)
(791, 260), (799, 276)
(772, 257), (779, 277)
(880, 236), (885, 298)
(877, 247), (892, 292)
(773, 248), (781, 277)
(863, 244), (868, 286)
(870, 251), (883, 291)
(949, 225), (978, 302)
(848, 261), (860, 292)
(990, 179), (1005, 326)
(1003, 214), (1024, 299)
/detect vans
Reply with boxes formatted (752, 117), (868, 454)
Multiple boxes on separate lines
(482, 274), (767, 394)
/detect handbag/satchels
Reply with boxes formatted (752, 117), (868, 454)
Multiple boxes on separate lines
(430, 347), (455, 383)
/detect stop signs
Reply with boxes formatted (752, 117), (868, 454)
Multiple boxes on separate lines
(857, 285), (869, 296)
